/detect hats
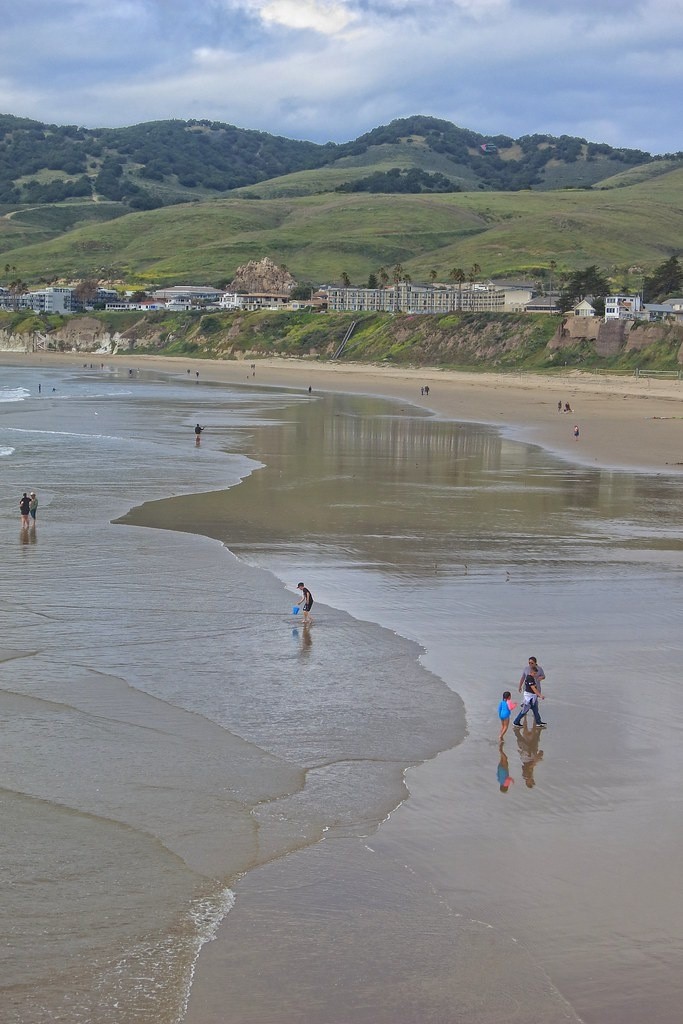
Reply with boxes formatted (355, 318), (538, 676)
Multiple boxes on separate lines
(297, 582), (304, 589)
(30, 492), (36, 495)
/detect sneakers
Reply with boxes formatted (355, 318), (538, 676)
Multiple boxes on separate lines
(512, 722), (524, 727)
(535, 722), (546, 727)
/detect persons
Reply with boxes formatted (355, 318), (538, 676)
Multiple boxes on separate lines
(297, 583), (313, 623)
(518, 657), (546, 706)
(558, 401), (571, 414)
(39, 363), (255, 394)
(20, 493), (31, 528)
(513, 667), (547, 727)
(498, 691), (517, 743)
(513, 727), (547, 788)
(29, 492), (38, 526)
(496, 743), (514, 793)
(574, 426), (579, 442)
(421, 385), (429, 395)
(195, 424), (204, 445)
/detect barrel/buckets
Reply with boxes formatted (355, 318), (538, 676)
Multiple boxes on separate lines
(292, 607), (299, 614)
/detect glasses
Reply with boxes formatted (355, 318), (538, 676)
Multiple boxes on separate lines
(529, 662), (535, 664)
